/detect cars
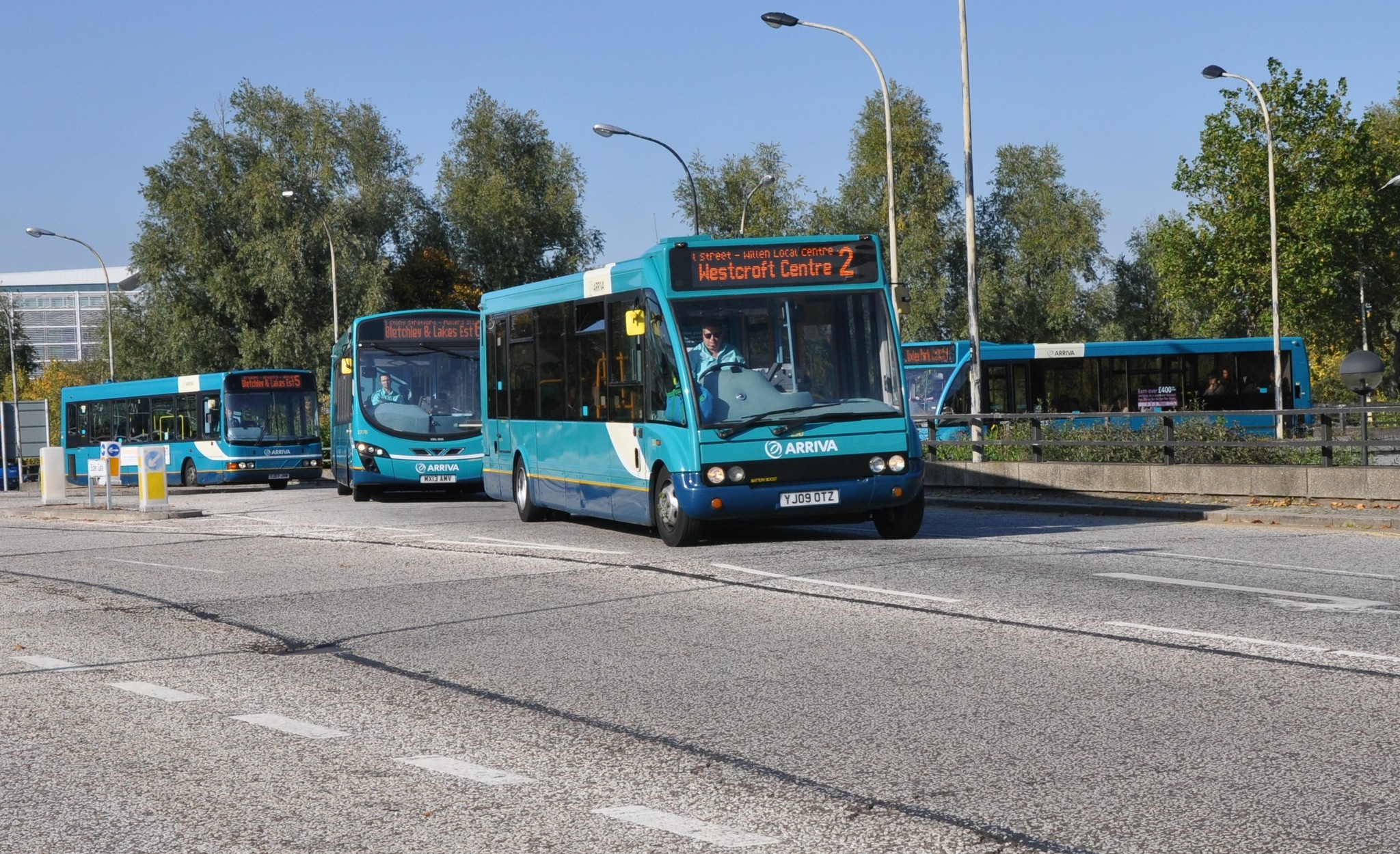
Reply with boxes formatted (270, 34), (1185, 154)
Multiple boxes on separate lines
(0, 457), (19, 491)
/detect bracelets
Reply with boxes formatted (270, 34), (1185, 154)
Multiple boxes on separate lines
(1212, 383), (1214, 385)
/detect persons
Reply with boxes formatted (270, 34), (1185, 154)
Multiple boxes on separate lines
(1218, 368), (1233, 384)
(217, 407), (240, 435)
(1256, 370), (1275, 394)
(371, 373), (406, 405)
(914, 377), (941, 402)
(687, 318), (747, 380)
(1203, 370), (1223, 395)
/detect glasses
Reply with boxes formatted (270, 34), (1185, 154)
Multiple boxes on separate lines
(704, 331), (721, 339)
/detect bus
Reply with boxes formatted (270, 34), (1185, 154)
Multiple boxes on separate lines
(476, 227), (927, 549)
(59, 367), (325, 492)
(900, 340), (1315, 462)
(327, 306), (497, 502)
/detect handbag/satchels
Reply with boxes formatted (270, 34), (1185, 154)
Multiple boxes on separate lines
(666, 383), (715, 424)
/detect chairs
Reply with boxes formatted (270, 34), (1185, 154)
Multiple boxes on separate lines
(80, 421), (126, 437)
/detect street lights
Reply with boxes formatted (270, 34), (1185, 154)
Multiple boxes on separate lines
(593, 123), (701, 237)
(738, 175), (776, 238)
(26, 226), (116, 384)
(760, 11), (900, 407)
(1200, 64), (1285, 441)
(280, 190), (340, 345)
